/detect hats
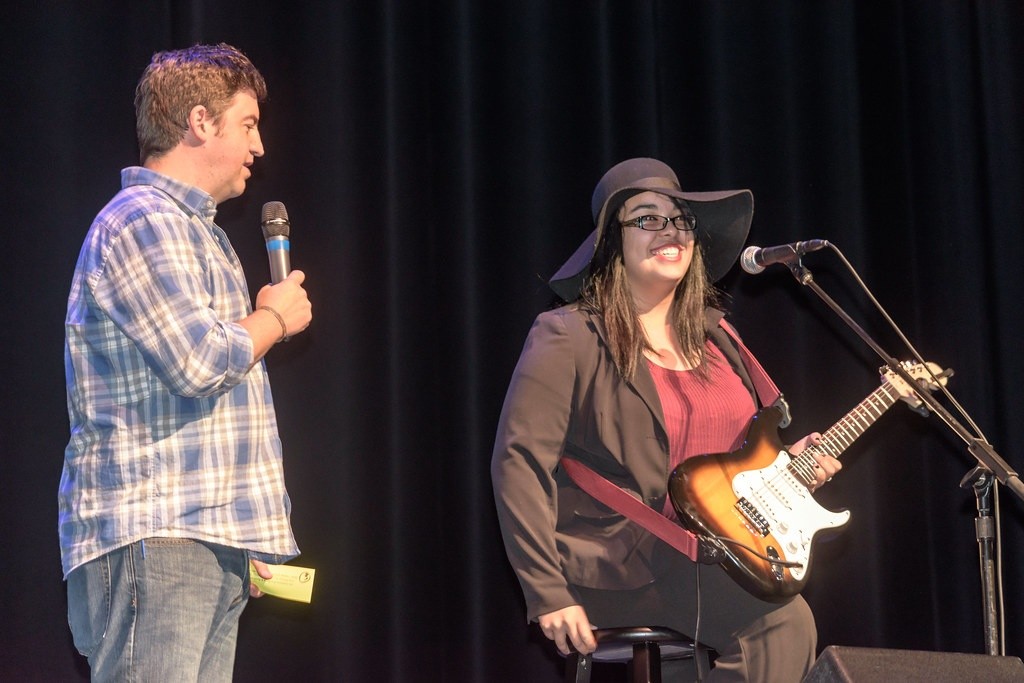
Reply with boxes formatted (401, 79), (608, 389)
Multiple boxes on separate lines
(547, 158), (752, 303)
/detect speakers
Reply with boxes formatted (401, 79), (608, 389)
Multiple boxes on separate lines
(802, 645), (1024, 683)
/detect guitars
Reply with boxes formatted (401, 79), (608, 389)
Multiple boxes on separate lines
(672, 358), (956, 606)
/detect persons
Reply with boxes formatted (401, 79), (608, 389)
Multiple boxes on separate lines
(490, 158), (842, 683)
(58, 44), (311, 683)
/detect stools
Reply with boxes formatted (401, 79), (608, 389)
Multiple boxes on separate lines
(557, 625), (715, 683)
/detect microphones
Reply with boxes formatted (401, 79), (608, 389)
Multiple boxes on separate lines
(741, 239), (828, 274)
(260, 202), (292, 342)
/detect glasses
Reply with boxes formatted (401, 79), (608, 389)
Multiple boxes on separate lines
(620, 212), (698, 231)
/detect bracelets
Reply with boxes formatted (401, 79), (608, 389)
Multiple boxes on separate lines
(256, 306), (286, 343)
